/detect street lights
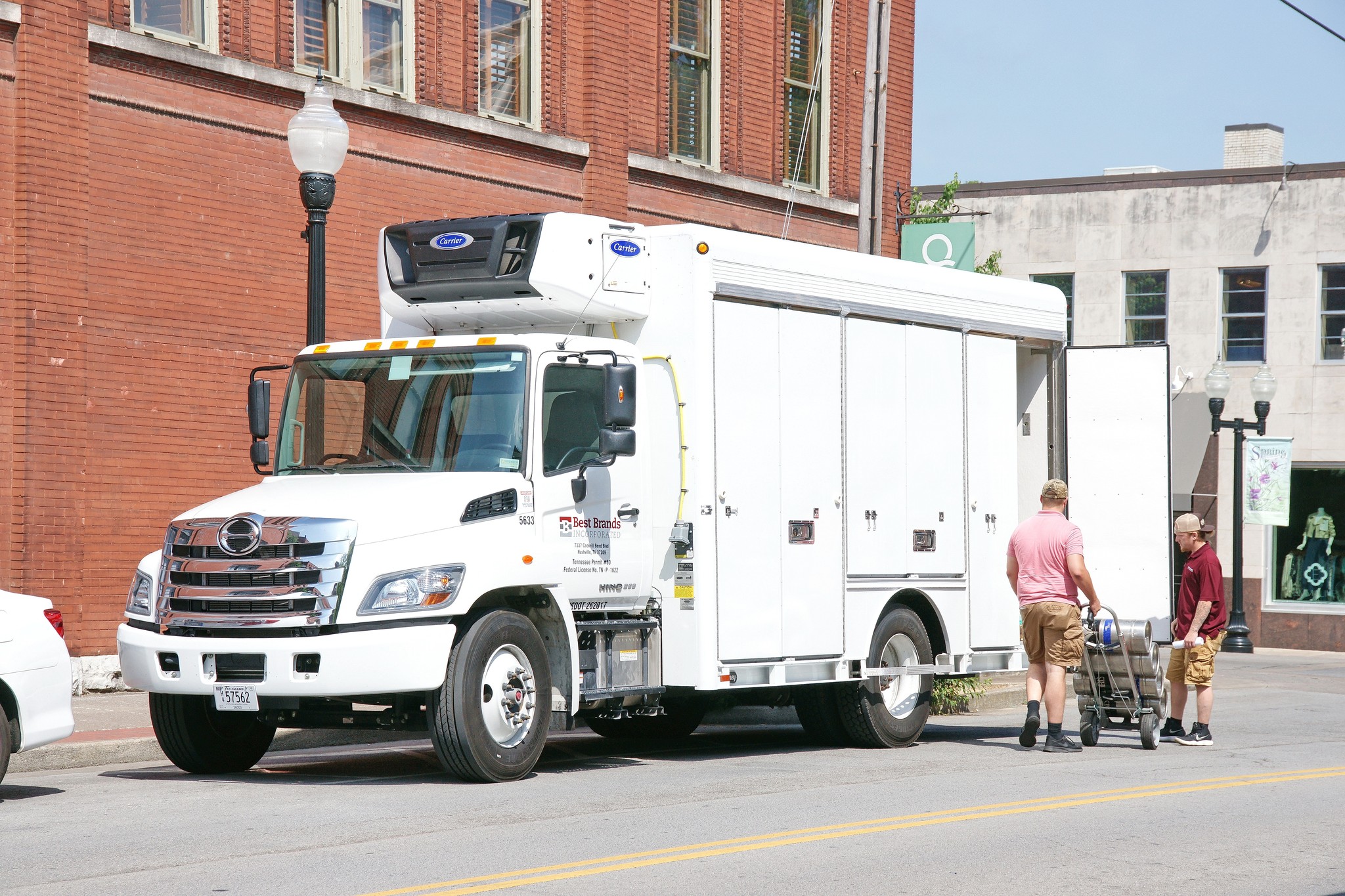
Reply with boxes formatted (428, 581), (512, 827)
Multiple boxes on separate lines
(1204, 351), (1278, 655)
(286, 62), (351, 472)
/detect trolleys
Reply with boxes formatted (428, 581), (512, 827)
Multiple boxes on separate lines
(1072, 600), (1169, 751)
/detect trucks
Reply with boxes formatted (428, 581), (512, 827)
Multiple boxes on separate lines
(111, 204), (1170, 787)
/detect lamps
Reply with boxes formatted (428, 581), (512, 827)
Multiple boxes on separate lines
(1278, 161), (1297, 191)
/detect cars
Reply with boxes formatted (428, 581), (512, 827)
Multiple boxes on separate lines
(1, 589), (75, 784)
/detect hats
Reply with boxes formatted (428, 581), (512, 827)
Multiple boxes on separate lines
(1042, 478), (1068, 499)
(1175, 513), (1215, 532)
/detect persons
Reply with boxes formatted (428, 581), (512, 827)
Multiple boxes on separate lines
(1157, 512), (1229, 746)
(1006, 477), (1101, 752)
(1297, 508), (1336, 601)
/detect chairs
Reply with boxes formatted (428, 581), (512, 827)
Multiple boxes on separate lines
(541, 389), (601, 472)
(406, 406), (458, 472)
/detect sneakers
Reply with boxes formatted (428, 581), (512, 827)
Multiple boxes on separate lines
(1174, 722), (1214, 746)
(1020, 710), (1042, 747)
(1043, 734), (1084, 753)
(1159, 717), (1186, 742)
